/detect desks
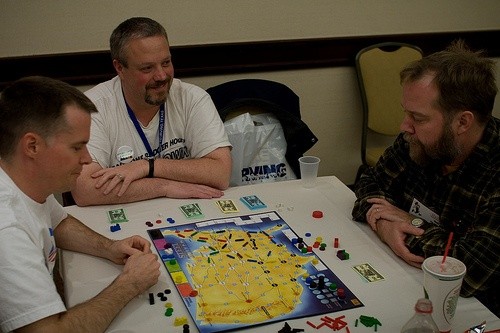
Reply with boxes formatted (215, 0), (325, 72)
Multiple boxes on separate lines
(57, 176), (500, 333)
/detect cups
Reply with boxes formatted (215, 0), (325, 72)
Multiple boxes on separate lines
(298, 156), (320, 188)
(421, 256), (467, 333)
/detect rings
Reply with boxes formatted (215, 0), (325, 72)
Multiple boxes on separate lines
(376, 208), (380, 213)
(117, 174), (123, 180)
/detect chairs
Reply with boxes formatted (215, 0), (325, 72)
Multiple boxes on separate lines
(355, 42), (423, 188)
(206, 78), (304, 182)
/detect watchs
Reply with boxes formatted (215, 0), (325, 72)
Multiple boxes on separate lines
(412, 218), (429, 229)
(143, 156), (157, 177)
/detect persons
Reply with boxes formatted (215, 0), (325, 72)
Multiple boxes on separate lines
(0, 76), (161, 333)
(74, 17), (233, 208)
(351, 41), (500, 320)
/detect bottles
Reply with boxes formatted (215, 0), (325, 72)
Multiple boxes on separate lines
(399, 299), (440, 333)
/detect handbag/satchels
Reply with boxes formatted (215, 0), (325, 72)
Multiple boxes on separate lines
(224, 111), (296, 187)
